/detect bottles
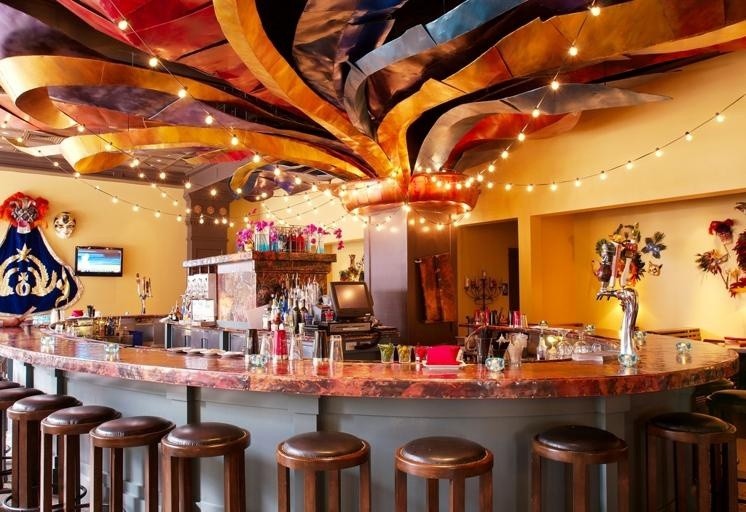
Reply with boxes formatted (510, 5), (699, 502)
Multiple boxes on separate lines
(260, 334), (272, 358)
(169, 294), (186, 320)
(261, 272), (333, 332)
(271, 228), (305, 253)
(296, 334), (303, 356)
(473, 306), (528, 329)
(536, 333), (547, 361)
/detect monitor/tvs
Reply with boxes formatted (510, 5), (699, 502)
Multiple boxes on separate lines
(74, 245), (124, 277)
(329, 281), (373, 322)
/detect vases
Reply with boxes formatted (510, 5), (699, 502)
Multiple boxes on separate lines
(316, 237), (325, 254)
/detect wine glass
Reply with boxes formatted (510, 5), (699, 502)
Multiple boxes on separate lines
(572, 330), (592, 353)
(556, 330), (572, 358)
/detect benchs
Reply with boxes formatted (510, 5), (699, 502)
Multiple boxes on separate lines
(650, 329), (701, 341)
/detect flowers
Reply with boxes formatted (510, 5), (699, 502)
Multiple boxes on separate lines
(303, 224), (345, 253)
(236, 209), (276, 250)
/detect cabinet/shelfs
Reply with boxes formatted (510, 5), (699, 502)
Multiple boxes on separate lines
(170, 325), (248, 354)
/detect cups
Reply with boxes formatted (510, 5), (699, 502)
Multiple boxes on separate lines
(54, 323), (63, 332)
(312, 330), (328, 363)
(379, 346), (396, 363)
(414, 346), (429, 363)
(254, 225), (271, 252)
(273, 330), (288, 359)
(307, 233), (317, 254)
(289, 329), (303, 360)
(329, 334), (344, 363)
(318, 236), (325, 254)
(397, 347), (412, 363)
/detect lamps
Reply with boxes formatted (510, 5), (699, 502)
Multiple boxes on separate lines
(464, 269), (508, 309)
